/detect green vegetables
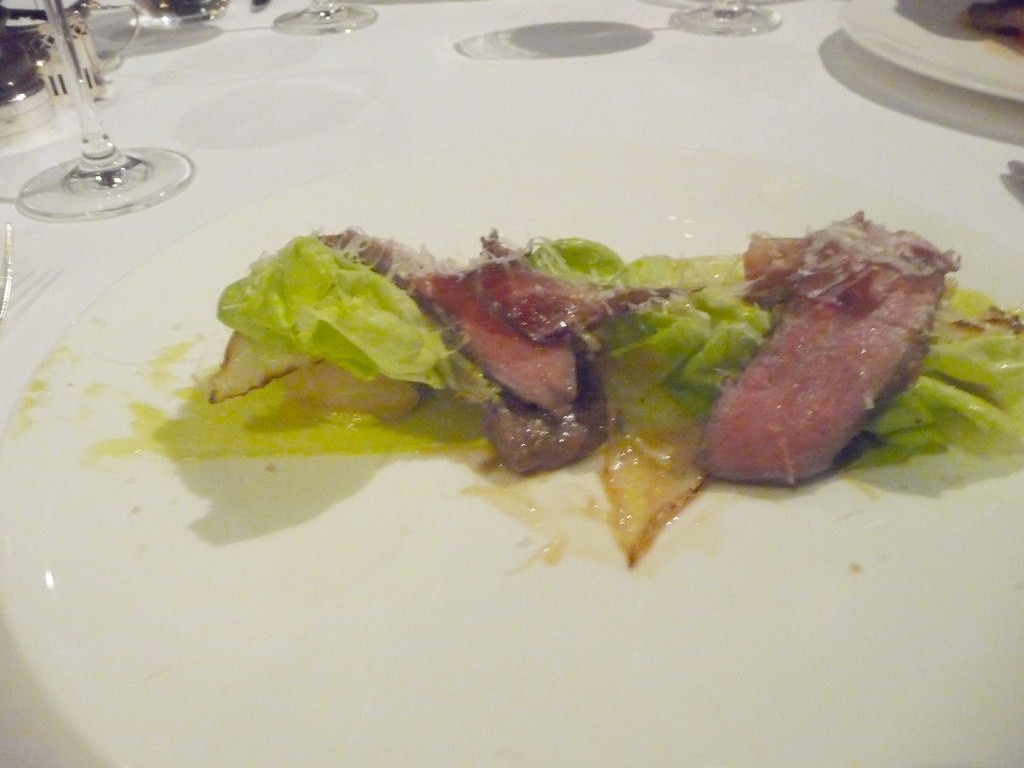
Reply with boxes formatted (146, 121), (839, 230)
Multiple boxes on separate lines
(216, 231), (1024, 468)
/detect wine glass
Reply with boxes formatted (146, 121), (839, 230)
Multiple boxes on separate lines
(14, 0), (194, 222)
(670, 0), (783, 36)
(272, 0), (377, 37)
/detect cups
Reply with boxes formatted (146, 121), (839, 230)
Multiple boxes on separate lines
(133, 0), (229, 25)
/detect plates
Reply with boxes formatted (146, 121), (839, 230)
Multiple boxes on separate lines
(835, 0), (1024, 105)
(0, 138), (1024, 768)
